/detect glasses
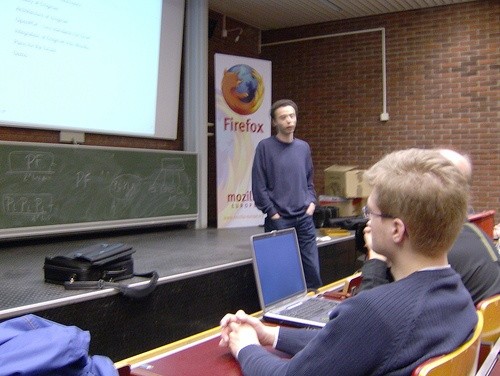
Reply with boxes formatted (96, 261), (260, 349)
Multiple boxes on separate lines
(363, 205), (393, 218)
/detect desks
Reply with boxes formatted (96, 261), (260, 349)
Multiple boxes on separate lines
(114, 270), (362, 376)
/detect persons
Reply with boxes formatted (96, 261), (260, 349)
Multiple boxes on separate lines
(218, 147), (500, 376)
(252, 100), (323, 289)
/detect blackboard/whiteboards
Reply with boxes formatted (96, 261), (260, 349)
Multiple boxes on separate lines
(0, 140), (200, 239)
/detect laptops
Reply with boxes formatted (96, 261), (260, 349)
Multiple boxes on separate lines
(250, 226), (342, 329)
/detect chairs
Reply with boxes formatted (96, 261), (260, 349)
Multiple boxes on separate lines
(410, 294), (500, 376)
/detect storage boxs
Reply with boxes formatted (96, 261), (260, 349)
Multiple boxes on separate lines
(318, 163), (368, 217)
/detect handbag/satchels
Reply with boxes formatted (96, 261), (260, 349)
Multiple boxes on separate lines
(43, 243), (136, 285)
